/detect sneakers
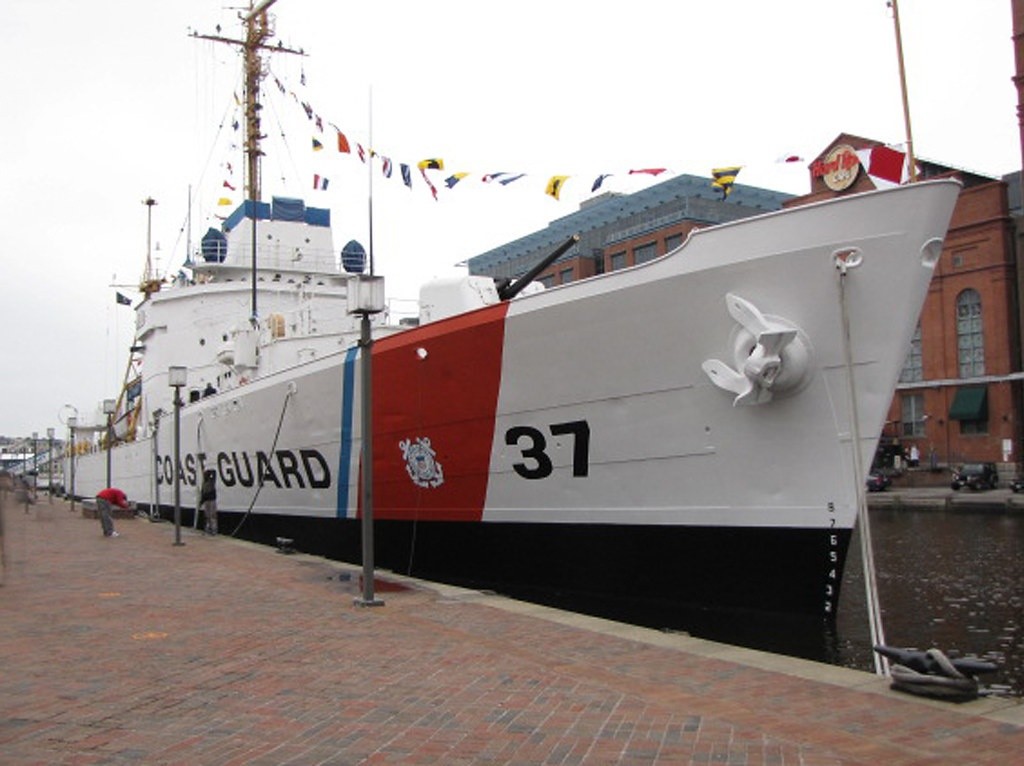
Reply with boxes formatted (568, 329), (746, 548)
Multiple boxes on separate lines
(107, 532), (118, 537)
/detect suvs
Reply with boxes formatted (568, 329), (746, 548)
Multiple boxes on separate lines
(950, 460), (999, 493)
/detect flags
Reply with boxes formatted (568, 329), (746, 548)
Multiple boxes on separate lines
(115, 292), (132, 305)
(274, 77), (920, 200)
(218, 94), (242, 207)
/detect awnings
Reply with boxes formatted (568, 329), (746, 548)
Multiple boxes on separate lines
(950, 388), (987, 421)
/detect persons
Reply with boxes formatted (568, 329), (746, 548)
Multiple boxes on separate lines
(203, 383), (216, 397)
(96, 487), (130, 538)
(903, 443), (919, 467)
(199, 471), (219, 536)
(178, 270), (186, 285)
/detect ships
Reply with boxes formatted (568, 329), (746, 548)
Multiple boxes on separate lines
(58, 3), (962, 665)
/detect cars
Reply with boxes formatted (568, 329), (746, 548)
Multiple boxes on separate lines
(866, 473), (892, 492)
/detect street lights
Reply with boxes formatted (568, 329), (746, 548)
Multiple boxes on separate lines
(102, 399), (116, 490)
(22, 446), (27, 490)
(67, 417), (78, 511)
(345, 273), (386, 606)
(47, 428), (55, 503)
(169, 366), (187, 547)
(33, 433), (39, 500)
